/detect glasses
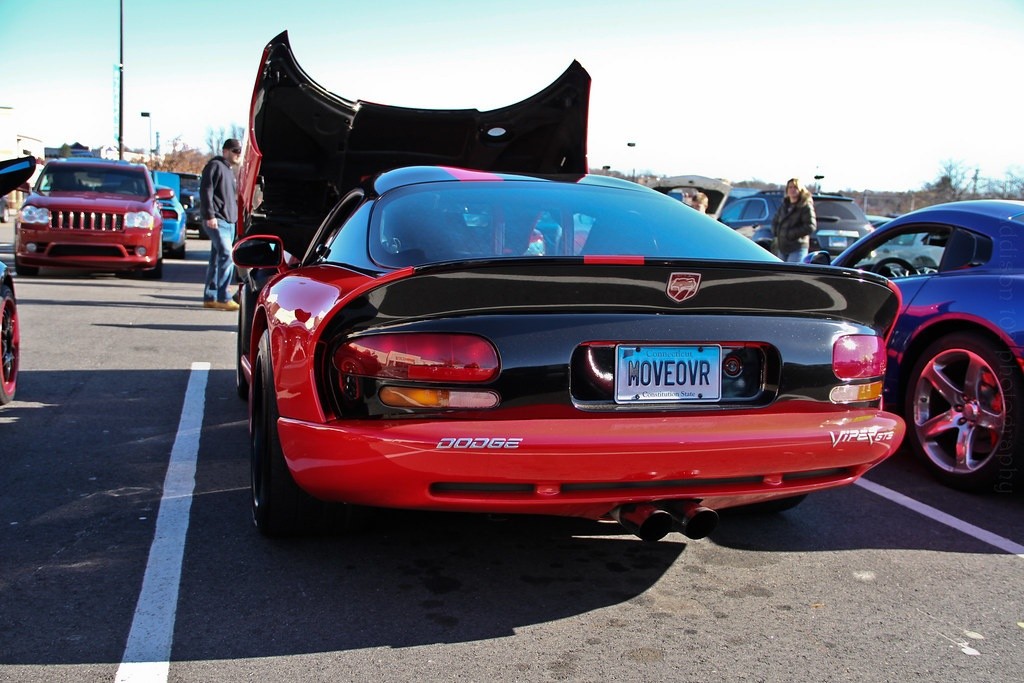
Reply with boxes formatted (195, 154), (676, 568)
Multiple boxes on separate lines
(232, 148), (241, 154)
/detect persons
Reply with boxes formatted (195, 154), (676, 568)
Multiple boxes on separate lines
(691, 192), (708, 214)
(773, 178), (817, 261)
(199, 139), (240, 310)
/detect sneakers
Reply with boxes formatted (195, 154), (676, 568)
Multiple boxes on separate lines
(217, 299), (240, 311)
(203, 300), (217, 307)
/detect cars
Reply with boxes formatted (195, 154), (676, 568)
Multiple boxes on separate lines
(154, 183), (187, 261)
(805, 196), (1024, 492)
(179, 171), (209, 240)
(12, 157), (163, 277)
(719, 188), (877, 263)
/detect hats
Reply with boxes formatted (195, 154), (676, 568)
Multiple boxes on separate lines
(222, 139), (243, 149)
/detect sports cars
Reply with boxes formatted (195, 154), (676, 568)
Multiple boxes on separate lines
(225, 27), (904, 545)
